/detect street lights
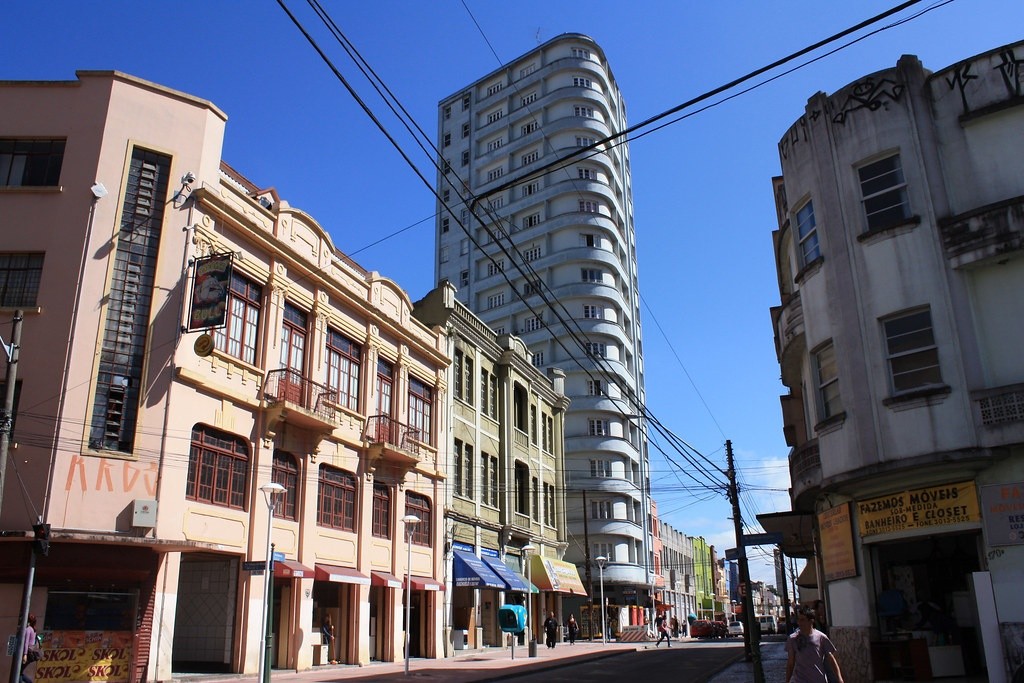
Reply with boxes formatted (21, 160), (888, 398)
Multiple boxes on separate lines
(521, 544), (541, 656)
(401, 514), (420, 676)
(594, 555), (612, 645)
(259, 482), (286, 683)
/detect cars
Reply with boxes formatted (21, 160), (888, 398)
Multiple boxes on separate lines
(753, 615), (777, 634)
(710, 620), (727, 637)
(726, 621), (744, 636)
(690, 619), (713, 639)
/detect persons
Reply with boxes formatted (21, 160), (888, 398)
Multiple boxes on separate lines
(544, 610), (560, 649)
(670, 613), (680, 638)
(566, 613), (579, 647)
(653, 613), (665, 641)
(807, 599), (832, 644)
(669, 615), (676, 638)
(789, 611), (797, 630)
(783, 606), (845, 682)
(681, 618), (688, 638)
(654, 615), (674, 648)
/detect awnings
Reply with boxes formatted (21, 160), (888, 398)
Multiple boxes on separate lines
(481, 554), (528, 594)
(454, 549), (507, 592)
(529, 552), (588, 599)
(511, 571), (540, 596)
